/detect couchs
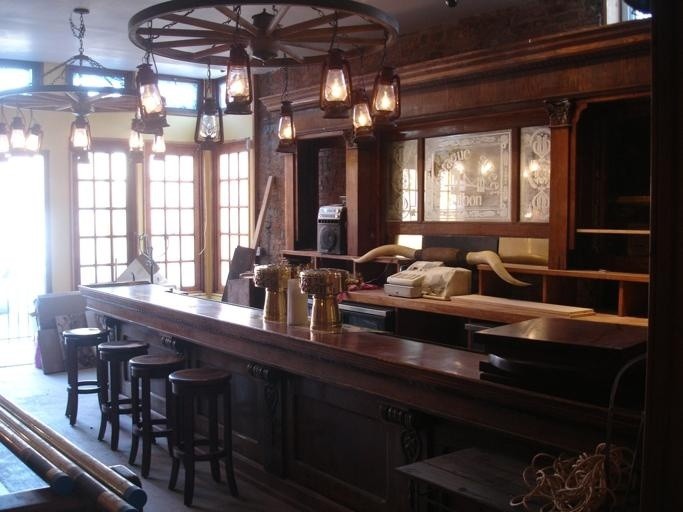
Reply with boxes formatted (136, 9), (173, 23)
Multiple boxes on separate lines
(30, 290), (98, 374)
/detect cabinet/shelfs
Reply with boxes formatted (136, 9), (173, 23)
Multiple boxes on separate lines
(258, 18), (650, 319)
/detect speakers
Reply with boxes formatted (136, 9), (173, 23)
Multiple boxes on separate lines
(317, 203), (347, 256)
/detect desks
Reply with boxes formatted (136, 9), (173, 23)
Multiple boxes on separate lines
(392, 447), (555, 511)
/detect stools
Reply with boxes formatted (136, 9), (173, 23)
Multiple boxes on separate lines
(62, 328), (107, 425)
(98, 340), (156, 450)
(168, 367), (239, 507)
(128, 355), (184, 477)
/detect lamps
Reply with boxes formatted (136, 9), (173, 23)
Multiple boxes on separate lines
(0, 8), (170, 159)
(127, 0), (405, 152)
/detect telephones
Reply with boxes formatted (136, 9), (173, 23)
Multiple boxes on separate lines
(387, 260), (444, 287)
